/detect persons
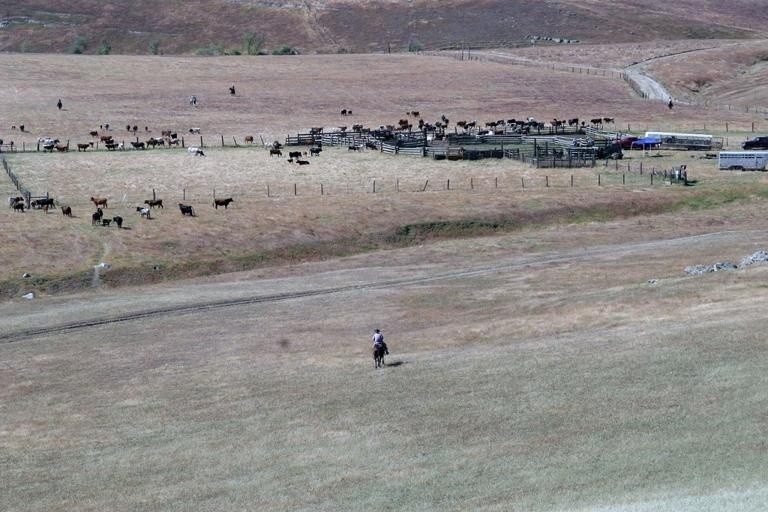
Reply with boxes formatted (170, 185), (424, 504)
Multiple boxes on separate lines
(372, 327), (389, 355)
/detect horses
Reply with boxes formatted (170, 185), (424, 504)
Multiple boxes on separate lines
(372, 342), (386, 369)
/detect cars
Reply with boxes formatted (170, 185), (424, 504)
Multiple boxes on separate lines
(612, 137), (637, 150)
(741, 136), (768, 150)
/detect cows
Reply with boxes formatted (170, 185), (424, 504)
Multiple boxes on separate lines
(270, 141), (323, 166)
(9, 195), (235, 230)
(378, 110), (614, 133)
(41, 122), (182, 152)
(188, 128), (206, 158)
(348, 142), (378, 151)
(244, 136), (254, 144)
